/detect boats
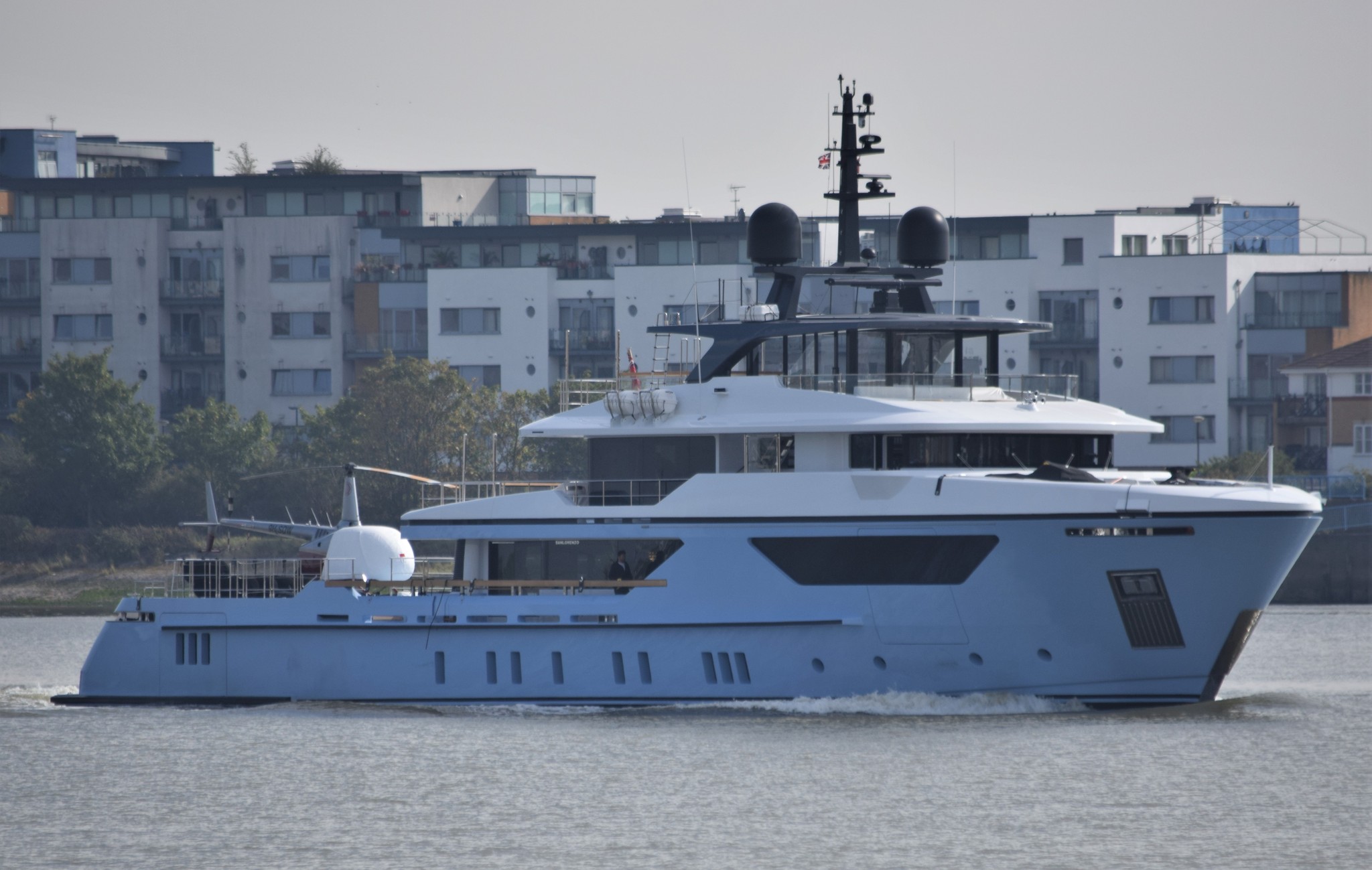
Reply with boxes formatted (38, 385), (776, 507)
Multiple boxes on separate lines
(76, 75), (1328, 709)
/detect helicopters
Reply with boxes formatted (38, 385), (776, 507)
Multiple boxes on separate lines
(175, 459), (459, 582)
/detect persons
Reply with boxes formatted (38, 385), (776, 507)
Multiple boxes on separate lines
(608, 550), (633, 594)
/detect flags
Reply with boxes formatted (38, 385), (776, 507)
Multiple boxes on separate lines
(818, 152), (831, 170)
